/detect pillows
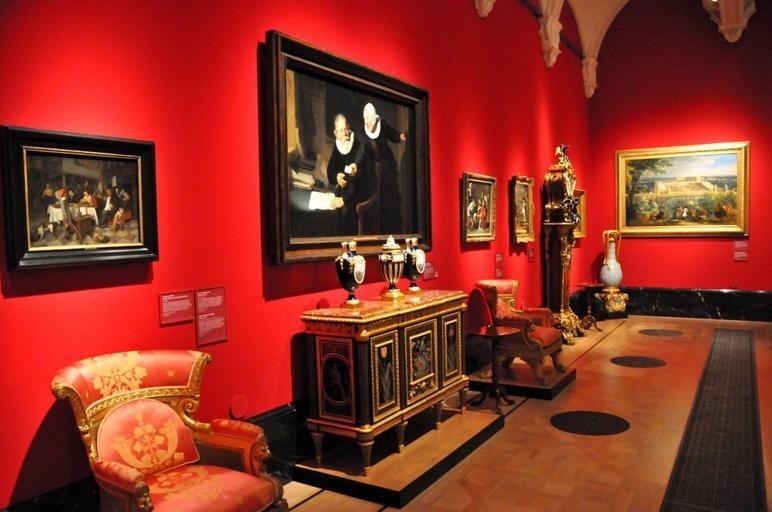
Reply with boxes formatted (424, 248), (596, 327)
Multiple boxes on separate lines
(97, 398), (200, 477)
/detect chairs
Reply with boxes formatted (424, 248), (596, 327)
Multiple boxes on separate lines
(50, 350), (288, 512)
(476, 279), (566, 385)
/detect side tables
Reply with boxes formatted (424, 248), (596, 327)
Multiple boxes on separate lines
(468, 326), (522, 415)
(575, 280), (607, 333)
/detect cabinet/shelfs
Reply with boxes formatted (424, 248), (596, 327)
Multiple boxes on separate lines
(301, 290), (468, 475)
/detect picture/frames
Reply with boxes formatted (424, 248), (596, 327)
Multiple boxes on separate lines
(463, 172), (496, 242)
(615, 141), (750, 237)
(573, 189), (587, 238)
(512, 175), (534, 243)
(0, 125), (158, 271)
(266, 30), (432, 266)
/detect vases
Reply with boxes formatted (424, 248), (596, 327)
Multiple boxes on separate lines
(599, 228), (623, 287)
(402, 237), (426, 292)
(378, 235), (404, 299)
(335, 240), (367, 307)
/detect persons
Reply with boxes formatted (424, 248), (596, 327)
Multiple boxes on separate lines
(31, 181), (131, 245)
(357, 101), (408, 234)
(466, 181), (490, 235)
(325, 112), (374, 236)
